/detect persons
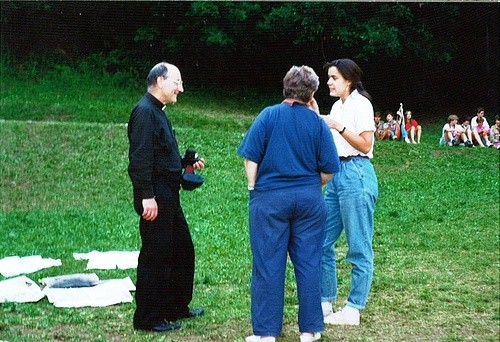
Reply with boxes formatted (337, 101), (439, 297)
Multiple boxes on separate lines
(374, 111), (422, 144)
(309, 59), (377, 325)
(237, 66), (340, 342)
(440, 109), (500, 149)
(128, 63), (204, 329)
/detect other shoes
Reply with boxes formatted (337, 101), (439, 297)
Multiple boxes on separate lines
(321, 302), (333, 316)
(464, 140), (473, 146)
(245, 335), (275, 342)
(323, 305), (361, 325)
(452, 138), (459, 145)
(153, 320), (181, 331)
(181, 307), (204, 317)
(486, 142), (493, 147)
(299, 331), (321, 342)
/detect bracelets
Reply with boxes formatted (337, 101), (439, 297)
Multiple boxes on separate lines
(339, 127), (346, 135)
